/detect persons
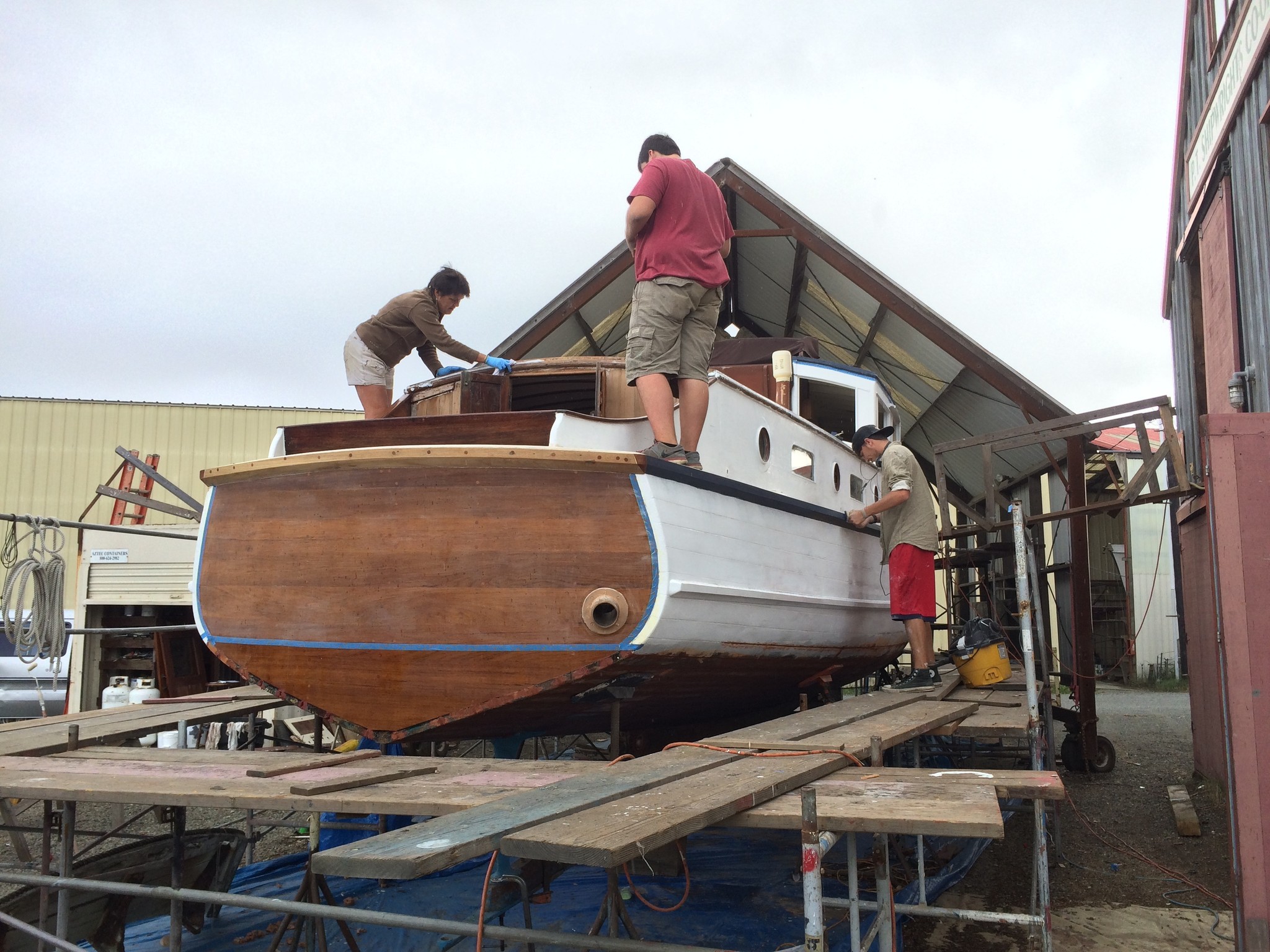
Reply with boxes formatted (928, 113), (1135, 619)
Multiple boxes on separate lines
(344, 268), (513, 420)
(625, 134), (736, 470)
(846, 424), (943, 694)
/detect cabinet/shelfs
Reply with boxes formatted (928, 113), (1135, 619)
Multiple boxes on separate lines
(96, 633), (157, 708)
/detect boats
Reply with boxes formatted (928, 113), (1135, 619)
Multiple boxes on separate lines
(188, 349), (910, 742)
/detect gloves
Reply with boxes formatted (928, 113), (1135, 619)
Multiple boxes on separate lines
(485, 355), (512, 373)
(436, 366), (467, 377)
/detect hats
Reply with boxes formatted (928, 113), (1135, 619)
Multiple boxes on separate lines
(852, 425), (895, 457)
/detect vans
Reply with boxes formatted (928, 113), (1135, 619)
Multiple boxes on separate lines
(0, 609), (74, 724)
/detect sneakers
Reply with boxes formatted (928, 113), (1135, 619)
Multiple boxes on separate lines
(681, 452), (703, 470)
(928, 663), (943, 687)
(635, 439), (688, 465)
(881, 668), (936, 693)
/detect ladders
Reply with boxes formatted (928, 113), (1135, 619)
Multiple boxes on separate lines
(108, 450), (160, 526)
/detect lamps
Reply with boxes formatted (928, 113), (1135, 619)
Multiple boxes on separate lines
(1227, 366), (1256, 409)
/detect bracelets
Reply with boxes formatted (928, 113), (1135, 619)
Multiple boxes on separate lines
(484, 355), (487, 363)
(861, 508), (869, 518)
(869, 514), (877, 524)
